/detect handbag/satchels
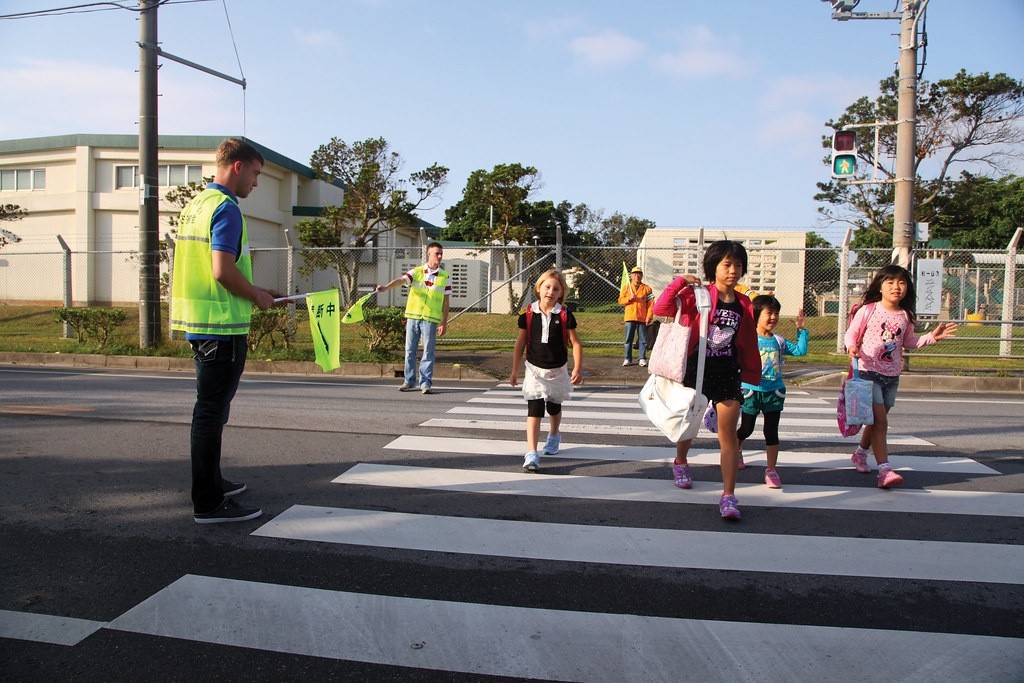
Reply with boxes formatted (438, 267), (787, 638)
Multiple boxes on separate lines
(837, 378), (863, 437)
(844, 358), (874, 424)
(648, 296), (691, 381)
(637, 374), (707, 443)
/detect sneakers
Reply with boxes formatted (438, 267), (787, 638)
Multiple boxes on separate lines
(671, 458), (693, 488)
(720, 495), (740, 519)
(738, 448), (746, 469)
(765, 468), (781, 488)
(422, 387), (431, 394)
(194, 501), (262, 523)
(222, 480), (246, 496)
(852, 451), (869, 472)
(877, 468), (902, 488)
(397, 381), (417, 390)
(523, 452), (540, 470)
(543, 431), (561, 455)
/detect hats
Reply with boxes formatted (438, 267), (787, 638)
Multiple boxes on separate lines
(631, 266), (643, 273)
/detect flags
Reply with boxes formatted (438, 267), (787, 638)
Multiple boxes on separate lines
(621, 263), (629, 287)
(342, 293), (372, 323)
(306, 289), (340, 371)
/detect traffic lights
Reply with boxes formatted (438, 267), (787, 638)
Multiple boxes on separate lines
(831, 131), (858, 178)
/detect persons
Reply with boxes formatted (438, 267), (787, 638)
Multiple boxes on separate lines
(734, 283), (773, 302)
(843, 265), (957, 488)
(509, 270), (583, 471)
(738, 295), (808, 489)
(618, 267), (655, 367)
(376, 242), (453, 394)
(168, 138), (294, 522)
(653, 240), (762, 519)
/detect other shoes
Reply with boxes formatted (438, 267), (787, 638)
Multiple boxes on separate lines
(640, 359), (647, 367)
(623, 359), (631, 367)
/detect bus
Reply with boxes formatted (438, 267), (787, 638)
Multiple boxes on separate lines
(808, 280), (866, 297)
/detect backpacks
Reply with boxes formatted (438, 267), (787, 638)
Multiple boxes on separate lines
(845, 302), (911, 354)
